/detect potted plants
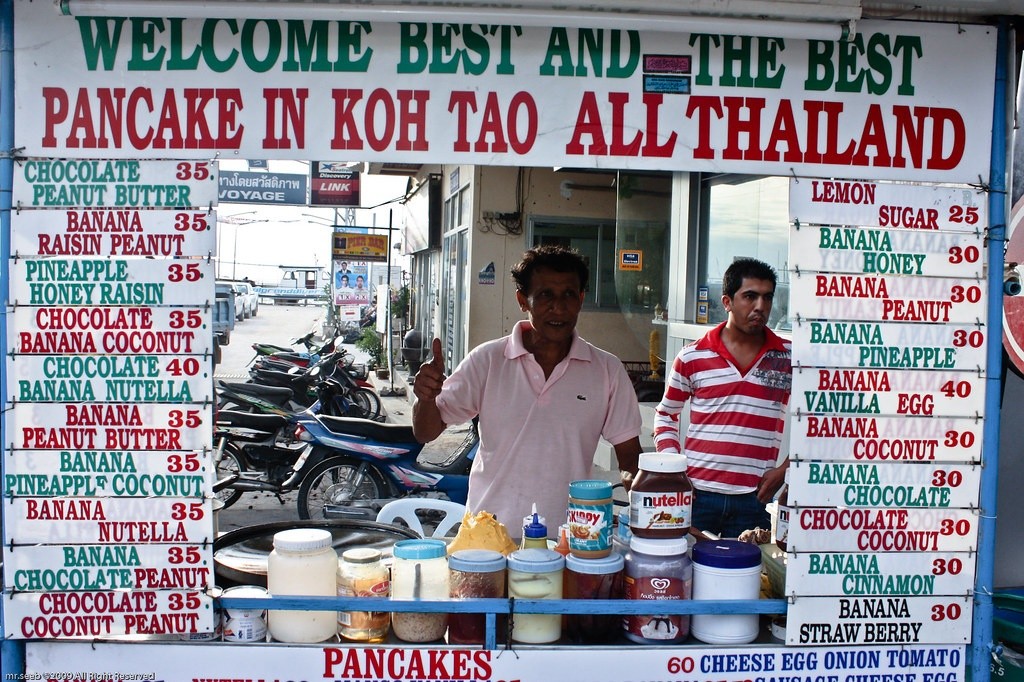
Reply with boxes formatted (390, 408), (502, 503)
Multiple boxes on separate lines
(356, 328), (388, 379)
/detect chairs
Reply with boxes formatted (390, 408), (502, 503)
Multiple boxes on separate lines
(376, 498), (466, 537)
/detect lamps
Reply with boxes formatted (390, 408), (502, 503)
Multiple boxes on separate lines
(53, 0), (864, 43)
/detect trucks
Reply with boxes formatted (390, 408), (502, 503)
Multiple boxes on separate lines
(210, 288), (236, 377)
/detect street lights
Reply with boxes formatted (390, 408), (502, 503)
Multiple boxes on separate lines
(218, 210), (258, 283)
(232, 219), (270, 281)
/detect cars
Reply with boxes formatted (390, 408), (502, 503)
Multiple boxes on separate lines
(214, 281), (259, 321)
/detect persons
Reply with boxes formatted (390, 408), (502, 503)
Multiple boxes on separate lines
(339, 275), (353, 291)
(338, 261), (351, 274)
(354, 275), (366, 290)
(412, 246), (644, 539)
(653, 258), (792, 539)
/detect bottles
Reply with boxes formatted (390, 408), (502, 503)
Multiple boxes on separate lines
(340, 538), (624, 646)
(266, 530), (338, 643)
(553, 529), (570, 554)
(522, 502), (546, 528)
(522, 513), (546, 550)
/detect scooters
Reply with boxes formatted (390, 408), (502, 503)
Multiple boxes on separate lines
(212, 328), (381, 511)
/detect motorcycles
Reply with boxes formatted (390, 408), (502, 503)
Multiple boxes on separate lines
(282, 404), (481, 532)
